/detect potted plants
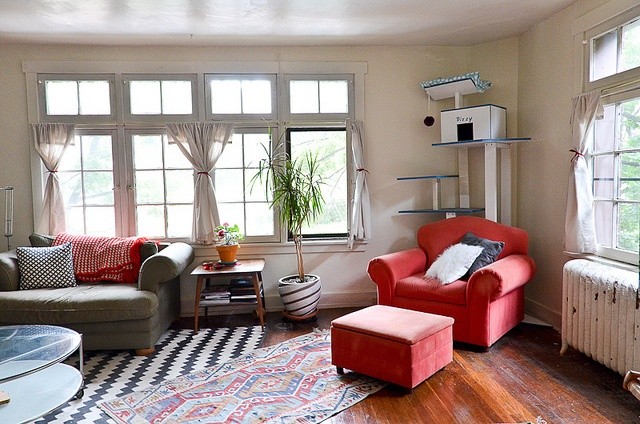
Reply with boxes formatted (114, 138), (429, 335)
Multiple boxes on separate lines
(251, 123), (333, 325)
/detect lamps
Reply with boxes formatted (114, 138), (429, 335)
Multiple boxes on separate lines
(1, 186), (13, 251)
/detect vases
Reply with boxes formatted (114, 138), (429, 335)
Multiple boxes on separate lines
(215, 245), (240, 265)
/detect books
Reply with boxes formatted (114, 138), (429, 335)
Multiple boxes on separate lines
(198, 285), (231, 305)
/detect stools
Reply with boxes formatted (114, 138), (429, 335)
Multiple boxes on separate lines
(329, 305), (455, 394)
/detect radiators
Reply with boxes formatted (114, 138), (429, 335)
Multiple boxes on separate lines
(557, 255), (640, 381)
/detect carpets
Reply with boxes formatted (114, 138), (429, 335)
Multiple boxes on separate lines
(94, 330), (387, 424)
(21, 326), (269, 423)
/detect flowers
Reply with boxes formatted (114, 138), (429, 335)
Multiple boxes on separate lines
(212, 222), (244, 249)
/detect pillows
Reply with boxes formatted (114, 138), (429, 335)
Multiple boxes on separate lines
(16, 245), (78, 290)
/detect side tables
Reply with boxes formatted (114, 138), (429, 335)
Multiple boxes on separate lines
(189, 257), (268, 334)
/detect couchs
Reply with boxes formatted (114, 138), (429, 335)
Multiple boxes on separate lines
(368, 214), (536, 352)
(15, 232), (194, 355)
